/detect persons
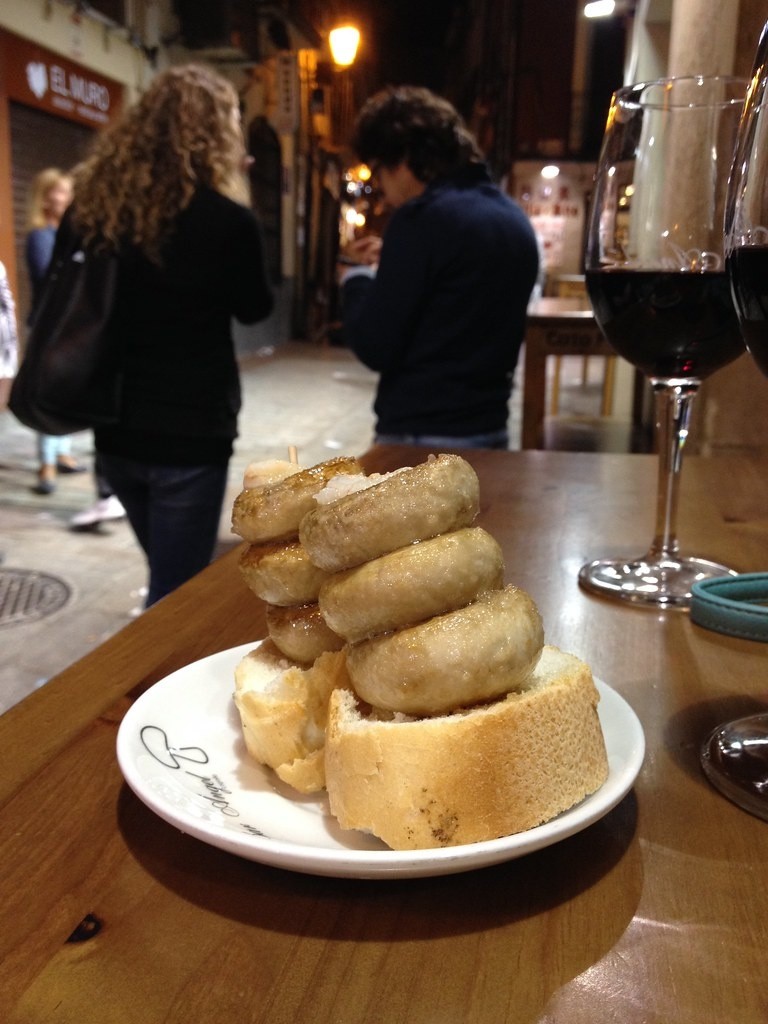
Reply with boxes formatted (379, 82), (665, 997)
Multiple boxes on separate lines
(26, 67), (280, 606)
(339, 90), (539, 449)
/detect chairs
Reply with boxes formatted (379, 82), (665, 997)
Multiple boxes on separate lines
(550, 273), (617, 417)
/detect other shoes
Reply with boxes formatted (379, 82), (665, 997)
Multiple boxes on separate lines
(38, 470), (56, 493)
(57, 461), (86, 473)
(70, 496), (125, 530)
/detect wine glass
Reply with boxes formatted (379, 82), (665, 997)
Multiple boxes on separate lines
(575, 72), (740, 610)
(697, 21), (768, 822)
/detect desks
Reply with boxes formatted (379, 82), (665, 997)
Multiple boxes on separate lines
(520, 298), (656, 453)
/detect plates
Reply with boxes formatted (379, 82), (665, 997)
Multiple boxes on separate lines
(114, 638), (646, 881)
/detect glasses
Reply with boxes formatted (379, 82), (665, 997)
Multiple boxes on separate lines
(358, 161), (384, 202)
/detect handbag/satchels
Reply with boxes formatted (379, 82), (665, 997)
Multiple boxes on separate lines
(6, 221), (120, 434)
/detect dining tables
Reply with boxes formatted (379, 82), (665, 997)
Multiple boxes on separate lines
(0, 443), (768, 1024)
